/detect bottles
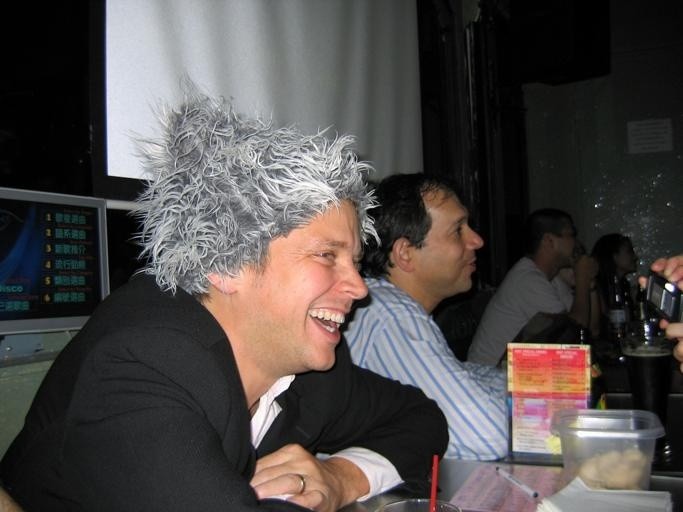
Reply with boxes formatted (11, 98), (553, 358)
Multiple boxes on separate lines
(606, 275), (649, 345)
(578, 327), (600, 411)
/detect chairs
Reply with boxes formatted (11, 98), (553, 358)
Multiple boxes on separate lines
(435, 297), (489, 361)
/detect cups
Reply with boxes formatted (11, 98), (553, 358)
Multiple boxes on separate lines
(617, 332), (681, 466)
(374, 498), (465, 512)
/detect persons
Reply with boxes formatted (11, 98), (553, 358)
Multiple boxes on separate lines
(0, 89), (452, 512)
(466, 206), (683, 408)
(338, 170), (511, 464)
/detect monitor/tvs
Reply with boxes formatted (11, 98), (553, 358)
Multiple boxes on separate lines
(0, 188), (111, 334)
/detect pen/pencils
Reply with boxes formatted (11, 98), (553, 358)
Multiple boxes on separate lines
(495, 466), (538, 498)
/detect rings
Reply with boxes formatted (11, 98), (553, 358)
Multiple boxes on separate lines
(294, 473), (306, 494)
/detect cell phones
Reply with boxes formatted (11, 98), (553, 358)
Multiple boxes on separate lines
(644, 274), (683, 324)
